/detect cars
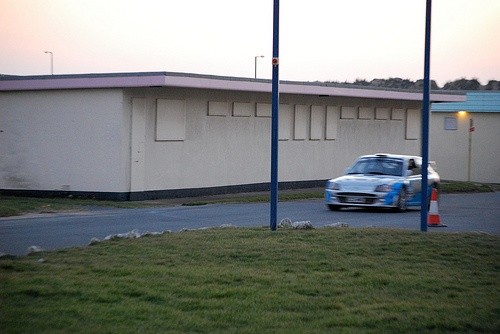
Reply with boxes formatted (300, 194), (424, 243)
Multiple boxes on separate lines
(325, 152), (440, 213)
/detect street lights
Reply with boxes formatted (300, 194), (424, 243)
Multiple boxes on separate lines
(255, 55), (264, 78)
(457, 109), (474, 182)
(44, 50), (53, 74)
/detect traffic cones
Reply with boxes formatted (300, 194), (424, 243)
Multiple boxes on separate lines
(427, 188), (447, 227)
